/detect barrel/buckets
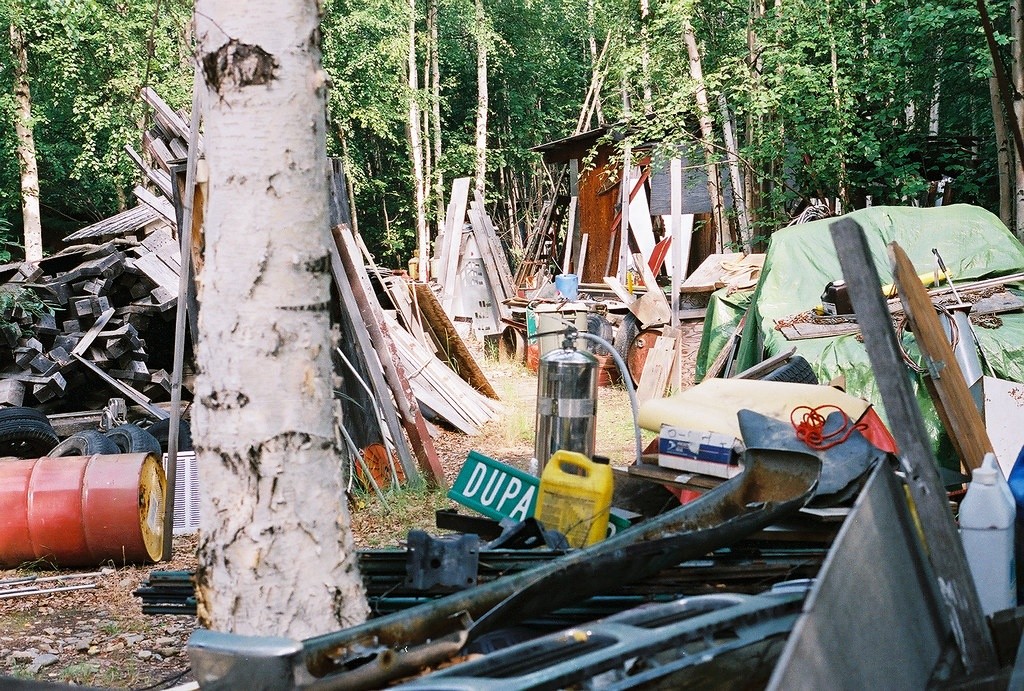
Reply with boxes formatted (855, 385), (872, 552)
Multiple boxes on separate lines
(627, 327), (663, 387)
(959, 452), (1017, 613)
(555, 274), (578, 301)
(0, 451), (166, 569)
(499, 325), (526, 366)
(533, 450), (614, 549)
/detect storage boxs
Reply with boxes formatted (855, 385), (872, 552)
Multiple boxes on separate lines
(655, 422), (748, 481)
